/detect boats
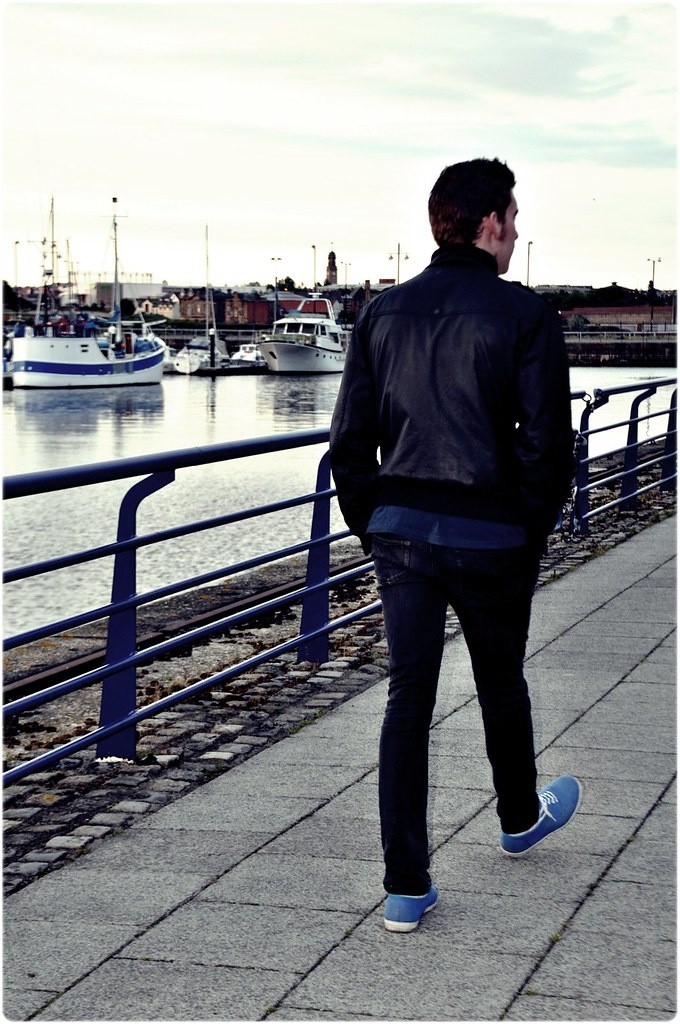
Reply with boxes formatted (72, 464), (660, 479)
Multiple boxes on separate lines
(255, 297), (348, 374)
(2, 195), (267, 388)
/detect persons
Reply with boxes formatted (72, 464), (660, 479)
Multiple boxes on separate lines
(329, 156), (582, 937)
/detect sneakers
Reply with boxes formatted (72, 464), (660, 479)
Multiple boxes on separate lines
(500, 775), (582, 857)
(383, 887), (437, 932)
